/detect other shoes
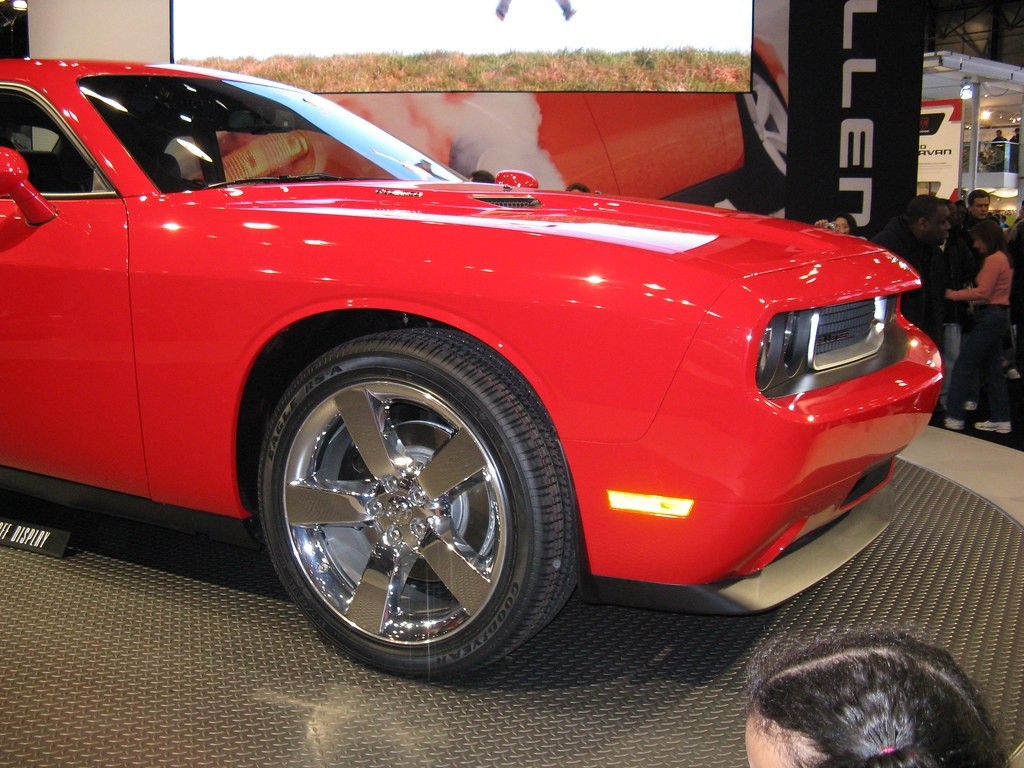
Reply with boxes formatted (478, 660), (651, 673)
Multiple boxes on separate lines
(1003, 368), (1021, 379)
(944, 417), (965, 430)
(975, 420), (1012, 433)
(963, 401), (978, 411)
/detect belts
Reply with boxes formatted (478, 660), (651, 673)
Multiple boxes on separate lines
(973, 304), (1009, 310)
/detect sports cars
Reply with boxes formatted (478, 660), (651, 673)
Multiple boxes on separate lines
(1, 54), (944, 678)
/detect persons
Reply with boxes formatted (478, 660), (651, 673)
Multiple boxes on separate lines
(564, 183), (591, 194)
(985, 128), (1022, 173)
(813, 190), (1023, 434)
(468, 170), (495, 184)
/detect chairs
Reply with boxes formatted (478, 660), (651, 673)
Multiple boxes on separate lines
(138, 154), (182, 192)
(19, 150), (81, 191)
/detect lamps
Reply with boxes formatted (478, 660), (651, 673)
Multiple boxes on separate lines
(959, 83), (972, 100)
